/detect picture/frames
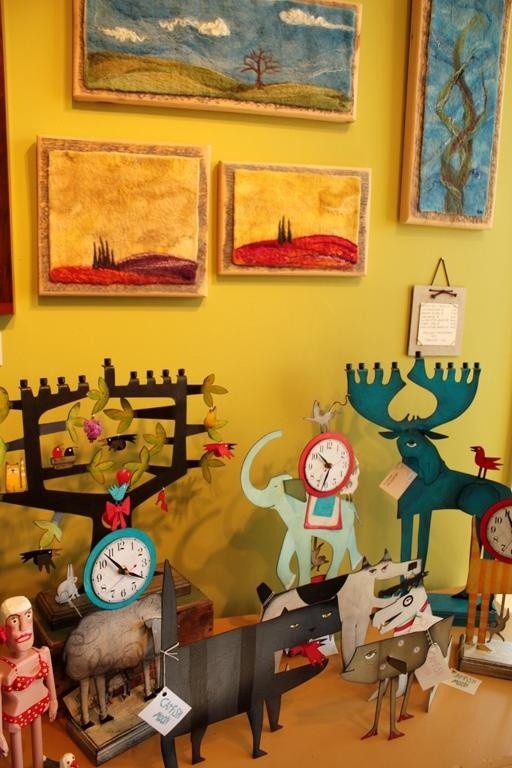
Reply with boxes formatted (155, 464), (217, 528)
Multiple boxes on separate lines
(69, 0), (360, 125)
(218, 163), (369, 275)
(398, 2), (511, 229)
(38, 138), (210, 299)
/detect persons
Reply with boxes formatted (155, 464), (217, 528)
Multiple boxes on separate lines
(1, 592), (75, 768)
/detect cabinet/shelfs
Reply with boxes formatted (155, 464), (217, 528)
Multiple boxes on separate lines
(30, 562), (229, 679)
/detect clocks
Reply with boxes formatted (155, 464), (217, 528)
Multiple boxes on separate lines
(480, 498), (512, 565)
(81, 528), (159, 610)
(302, 430), (354, 495)
